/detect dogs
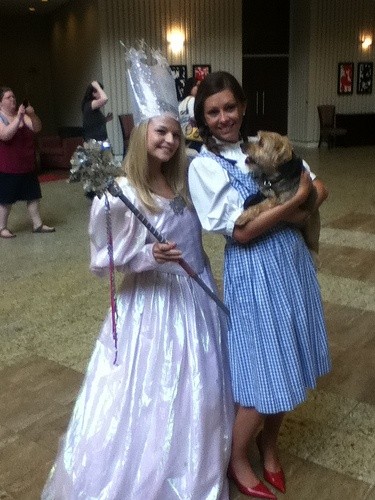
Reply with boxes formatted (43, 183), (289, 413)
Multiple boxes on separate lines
(242, 132), (323, 269)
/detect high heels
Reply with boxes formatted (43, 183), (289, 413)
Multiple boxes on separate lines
(256, 445), (286, 494)
(226, 459), (278, 500)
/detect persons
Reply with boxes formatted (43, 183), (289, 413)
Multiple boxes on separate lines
(179, 79), (204, 153)
(42, 101), (235, 500)
(0, 87), (56, 238)
(188, 69), (330, 500)
(81, 81), (113, 142)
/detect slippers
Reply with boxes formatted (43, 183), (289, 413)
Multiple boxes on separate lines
(0, 227), (16, 238)
(32, 224), (55, 233)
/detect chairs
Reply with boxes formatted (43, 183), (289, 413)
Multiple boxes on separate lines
(318, 105), (347, 151)
(119, 114), (135, 160)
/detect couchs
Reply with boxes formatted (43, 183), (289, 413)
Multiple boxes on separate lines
(41, 127), (83, 173)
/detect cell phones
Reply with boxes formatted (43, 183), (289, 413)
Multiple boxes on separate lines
(23, 99), (28, 108)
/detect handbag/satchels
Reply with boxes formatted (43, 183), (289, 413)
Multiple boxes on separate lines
(184, 117), (203, 143)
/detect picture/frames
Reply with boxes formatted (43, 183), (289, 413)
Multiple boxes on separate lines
(193, 65), (211, 81)
(357, 62), (373, 94)
(170, 65), (187, 81)
(338, 62), (354, 94)
